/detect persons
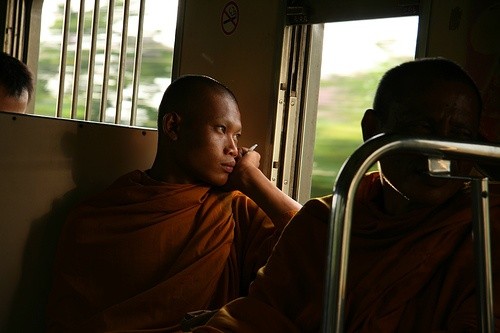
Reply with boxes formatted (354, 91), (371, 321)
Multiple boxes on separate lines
(43, 74), (303, 333)
(192, 58), (500, 333)
(0, 52), (37, 115)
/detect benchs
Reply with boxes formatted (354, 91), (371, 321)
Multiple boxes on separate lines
(0, 110), (158, 321)
